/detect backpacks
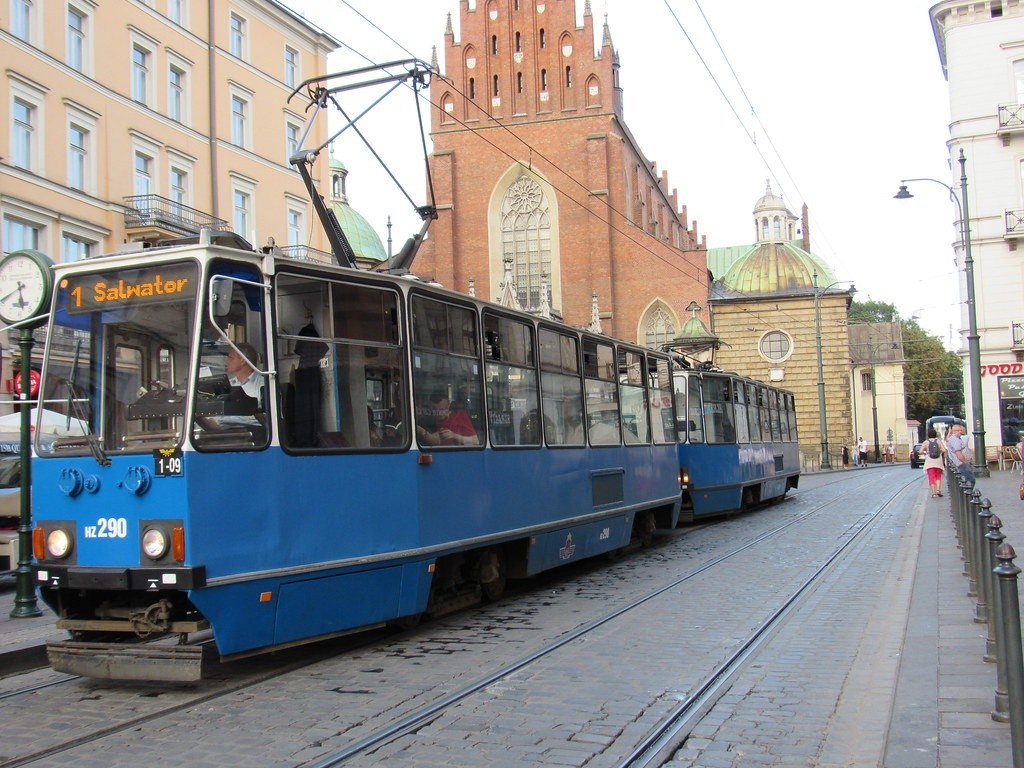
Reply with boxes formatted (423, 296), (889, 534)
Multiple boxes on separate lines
(928, 437), (940, 459)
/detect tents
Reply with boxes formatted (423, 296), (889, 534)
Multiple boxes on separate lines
(0, 405), (93, 453)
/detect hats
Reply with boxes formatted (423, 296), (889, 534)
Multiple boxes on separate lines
(858, 437), (862, 440)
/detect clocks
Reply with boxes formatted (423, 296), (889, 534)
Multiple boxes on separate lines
(0, 252), (53, 328)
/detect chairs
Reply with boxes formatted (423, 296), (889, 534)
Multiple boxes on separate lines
(985, 445), (1024, 473)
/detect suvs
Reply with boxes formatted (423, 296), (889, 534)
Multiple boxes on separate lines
(910, 444), (925, 469)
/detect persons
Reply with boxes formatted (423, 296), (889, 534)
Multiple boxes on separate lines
(1012, 435), (1023, 477)
(361, 381), (479, 447)
(881, 443), (895, 464)
(841, 437), (869, 467)
(919, 425), (976, 497)
(219, 345), (265, 416)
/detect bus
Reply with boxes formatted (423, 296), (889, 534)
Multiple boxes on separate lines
(925, 415), (967, 443)
(28, 57), (801, 686)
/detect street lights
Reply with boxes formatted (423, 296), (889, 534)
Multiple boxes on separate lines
(812, 268), (860, 472)
(892, 147), (990, 479)
(869, 338), (900, 464)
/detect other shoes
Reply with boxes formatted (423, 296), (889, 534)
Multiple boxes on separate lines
(865, 464), (867, 467)
(936, 490), (944, 497)
(862, 465), (864, 467)
(930, 493), (936, 498)
